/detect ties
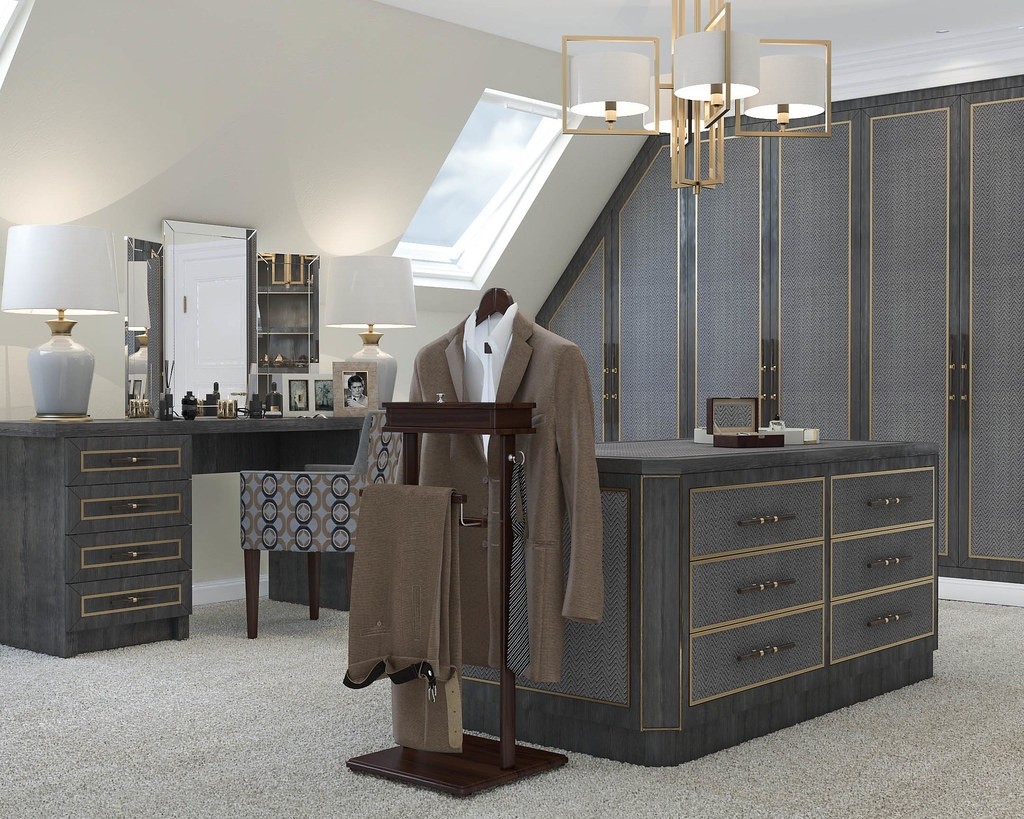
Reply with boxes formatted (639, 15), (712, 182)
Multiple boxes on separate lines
(507, 461), (531, 680)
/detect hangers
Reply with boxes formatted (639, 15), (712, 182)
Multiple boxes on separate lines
(474, 286), (513, 325)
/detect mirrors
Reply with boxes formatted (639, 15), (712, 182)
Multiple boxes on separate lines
(123, 235), (164, 419)
(256, 251), (319, 373)
(161, 218), (248, 414)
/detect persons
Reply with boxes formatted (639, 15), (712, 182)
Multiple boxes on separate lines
(344, 375), (369, 408)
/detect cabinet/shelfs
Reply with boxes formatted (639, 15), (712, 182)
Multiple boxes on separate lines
(462, 440), (941, 767)
(257, 290), (318, 373)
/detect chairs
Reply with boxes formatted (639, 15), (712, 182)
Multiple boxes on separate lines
(240, 410), (403, 639)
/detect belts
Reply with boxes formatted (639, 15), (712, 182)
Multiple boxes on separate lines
(342, 658), (439, 704)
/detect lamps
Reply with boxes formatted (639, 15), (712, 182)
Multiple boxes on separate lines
(324, 255), (417, 411)
(560, 0), (831, 195)
(128, 260), (151, 373)
(1, 225), (121, 427)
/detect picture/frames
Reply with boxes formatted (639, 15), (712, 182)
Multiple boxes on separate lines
(332, 361), (380, 417)
(282, 373), (335, 417)
(129, 373), (147, 400)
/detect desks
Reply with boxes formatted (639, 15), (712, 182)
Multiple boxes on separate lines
(0, 417), (363, 659)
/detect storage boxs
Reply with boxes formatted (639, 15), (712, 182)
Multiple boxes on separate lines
(708, 399), (786, 448)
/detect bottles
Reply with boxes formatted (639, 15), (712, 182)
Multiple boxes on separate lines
(250, 394), (262, 418)
(266, 382), (283, 418)
(182, 391), (198, 420)
